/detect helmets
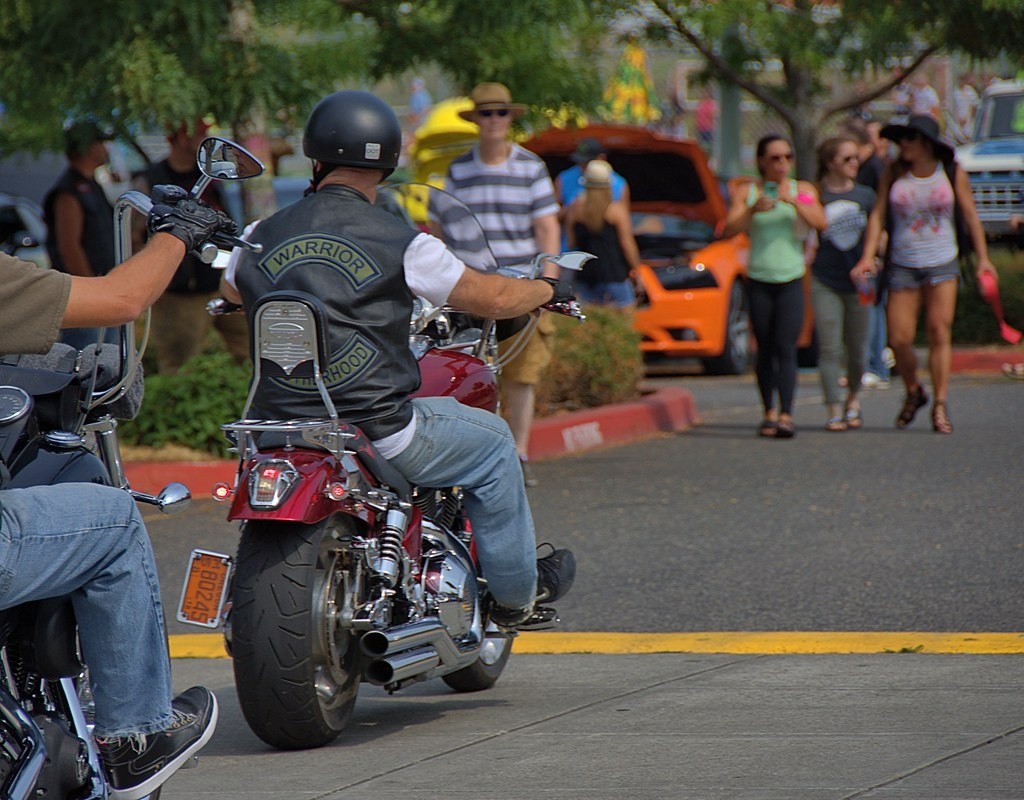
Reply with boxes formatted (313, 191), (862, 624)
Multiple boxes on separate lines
(302, 91), (401, 169)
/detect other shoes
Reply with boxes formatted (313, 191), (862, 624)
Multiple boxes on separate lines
(860, 373), (890, 391)
(877, 344), (896, 369)
(836, 376), (849, 388)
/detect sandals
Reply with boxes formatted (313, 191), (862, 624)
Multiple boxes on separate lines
(760, 418), (778, 438)
(897, 385), (927, 428)
(931, 399), (952, 434)
(774, 414), (794, 436)
(844, 397), (863, 427)
(824, 410), (847, 431)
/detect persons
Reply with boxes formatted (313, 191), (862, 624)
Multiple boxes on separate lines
(723, 69), (995, 439)
(1, 199), (218, 799)
(218, 87), (577, 627)
(556, 159), (660, 399)
(426, 82), (560, 488)
(232, 146), (261, 177)
(553, 138), (631, 263)
(39, 115), (121, 351)
(129, 116), (233, 374)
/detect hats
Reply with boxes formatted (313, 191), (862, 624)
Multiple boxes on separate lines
(458, 81), (529, 125)
(578, 160), (617, 188)
(163, 113), (216, 144)
(66, 121), (114, 156)
(878, 116), (954, 164)
(570, 138), (602, 164)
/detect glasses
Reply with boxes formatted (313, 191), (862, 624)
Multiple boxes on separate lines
(480, 109), (510, 117)
(771, 155), (792, 161)
(844, 156), (859, 163)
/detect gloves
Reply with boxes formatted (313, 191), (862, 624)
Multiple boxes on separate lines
(536, 275), (575, 316)
(157, 200), (227, 257)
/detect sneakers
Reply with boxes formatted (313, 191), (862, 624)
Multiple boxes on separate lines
(487, 543), (576, 627)
(95, 685), (218, 800)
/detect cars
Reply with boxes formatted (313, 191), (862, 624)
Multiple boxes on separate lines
(517, 118), (819, 376)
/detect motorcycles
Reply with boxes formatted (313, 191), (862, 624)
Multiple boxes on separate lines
(0, 138), (268, 800)
(177, 180), (603, 749)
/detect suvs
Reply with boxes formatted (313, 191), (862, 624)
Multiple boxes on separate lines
(951, 77), (1024, 247)
